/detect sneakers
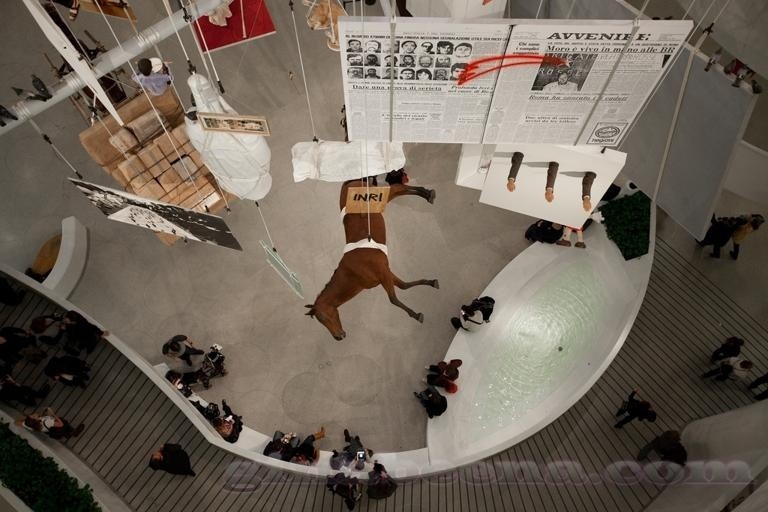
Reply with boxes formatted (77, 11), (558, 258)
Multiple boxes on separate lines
(344, 429), (351, 442)
(355, 435), (359, 440)
(413, 365), (430, 399)
(73, 423), (85, 438)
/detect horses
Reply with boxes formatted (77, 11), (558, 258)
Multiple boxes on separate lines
(305, 176), (440, 341)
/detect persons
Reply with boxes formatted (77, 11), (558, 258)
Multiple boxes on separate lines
(412, 385), (450, 423)
(636, 428), (688, 466)
(423, 362), (459, 390)
(346, 37), (471, 80)
(541, 69), (576, 92)
(261, 425), (398, 510)
(729, 213), (765, 260)
(544, 162), (559, 203)
(0, 309), (109, 439)
(581, 172), (596, 212)
(694, 212), (745, 261)
(507, 151), (524, 192)
(613, 386), (656, 430)
(699, 336), (768, 402)
(153, 333), (243, 444)
(149, 444), (197, 477)
(127, 59), (173, 97)
(451, 304), (483, 334)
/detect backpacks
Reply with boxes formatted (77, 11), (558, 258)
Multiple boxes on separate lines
(719, 359), (745, 376)
(177, 383), (192, 398)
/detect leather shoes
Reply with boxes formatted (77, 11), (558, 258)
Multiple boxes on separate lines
(186, 350), (204, 366)
(222, 399), (227, 411)
(695, 239), (739, 260)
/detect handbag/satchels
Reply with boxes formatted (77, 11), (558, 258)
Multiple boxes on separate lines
(43, 419), (62, 440)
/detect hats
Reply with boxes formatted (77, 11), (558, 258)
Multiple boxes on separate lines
(211, 343), (223, 351)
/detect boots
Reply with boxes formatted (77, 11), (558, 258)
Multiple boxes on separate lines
(312, 426), (326, 441)
